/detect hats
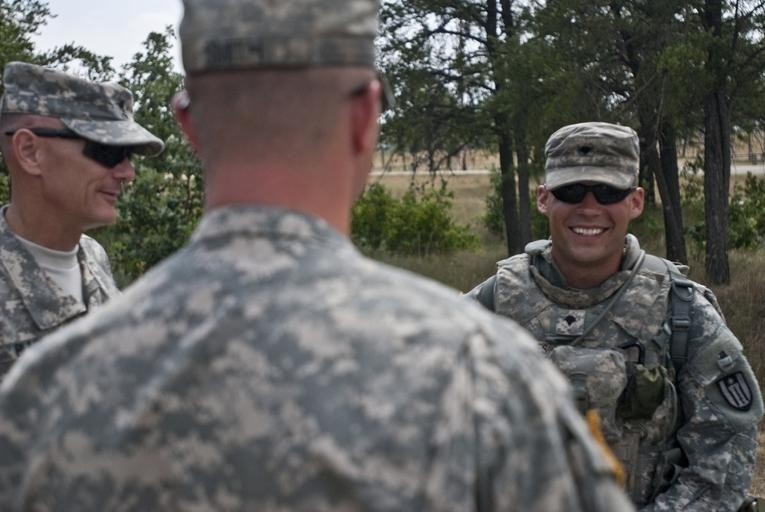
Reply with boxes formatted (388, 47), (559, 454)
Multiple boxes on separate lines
(180, 2), (380, 73)
(0, 61), (165, 159)
(545, 122), (641, 191)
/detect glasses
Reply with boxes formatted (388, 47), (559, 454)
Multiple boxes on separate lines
(550, 183), (637, 205)
(4, 127), (134, 169)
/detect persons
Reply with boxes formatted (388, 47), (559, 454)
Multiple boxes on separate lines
(0, 0), (639, 512)
(464, 120), (765, 512)
(1, 61), (166, 380)
